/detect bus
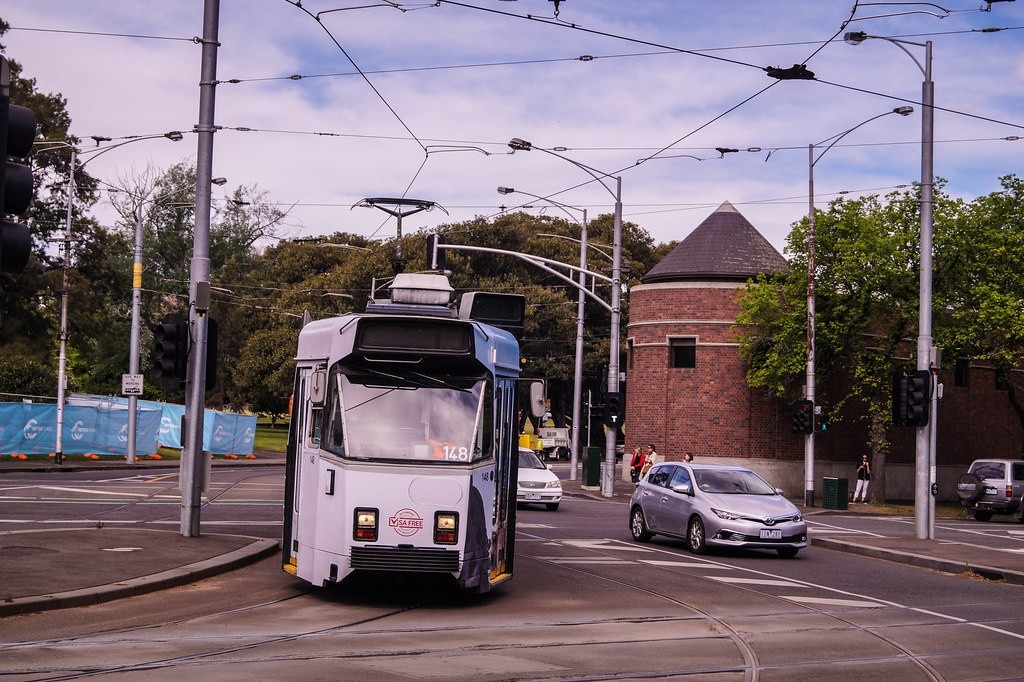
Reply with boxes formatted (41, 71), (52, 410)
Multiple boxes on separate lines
(278, 197), (549, 604)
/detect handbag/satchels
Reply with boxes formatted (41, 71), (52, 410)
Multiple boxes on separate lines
(642, 462), (651, 474)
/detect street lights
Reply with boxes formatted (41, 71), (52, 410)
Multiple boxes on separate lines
(842, 30), (935, 542)
(125, 177), (227, 465)
(497, 186), (589, 482)
(52, 131), (185, 465)
(806, 104), (914, 516)
(509, 137), (625, 498)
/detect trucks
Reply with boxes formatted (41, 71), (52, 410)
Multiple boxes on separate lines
(533, 427), (572, 462)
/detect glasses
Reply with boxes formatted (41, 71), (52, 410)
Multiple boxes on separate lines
(863, 458), (867, 460)
(647, 446), (651, 449)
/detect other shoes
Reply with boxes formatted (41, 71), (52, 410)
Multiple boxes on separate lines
(862, 501), (867, 504)
(849, 501), (856, 504)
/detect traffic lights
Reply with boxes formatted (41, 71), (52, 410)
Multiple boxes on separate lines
(604, 391), (625, 428)
(815, 413), (830, 433)
(150, 313), (186, 391)
(905, 369), (930, 427)
(0, 53), (38, 280)
(791, 400), (814, 435)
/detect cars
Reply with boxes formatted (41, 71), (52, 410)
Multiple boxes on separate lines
(516, 445), (564, 511)
(626, 460), (809, 560)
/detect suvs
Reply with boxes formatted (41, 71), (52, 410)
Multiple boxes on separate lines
(956, 458), (1024, 524)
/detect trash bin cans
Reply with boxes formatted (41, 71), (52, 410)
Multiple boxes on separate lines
(823, 477), (849, 510)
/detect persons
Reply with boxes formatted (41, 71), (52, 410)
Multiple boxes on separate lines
(849, 454), (871, 505)
(629, 448), (646, 488)
(682, 452), (694, 463)
(644, 444), (657, 466)
